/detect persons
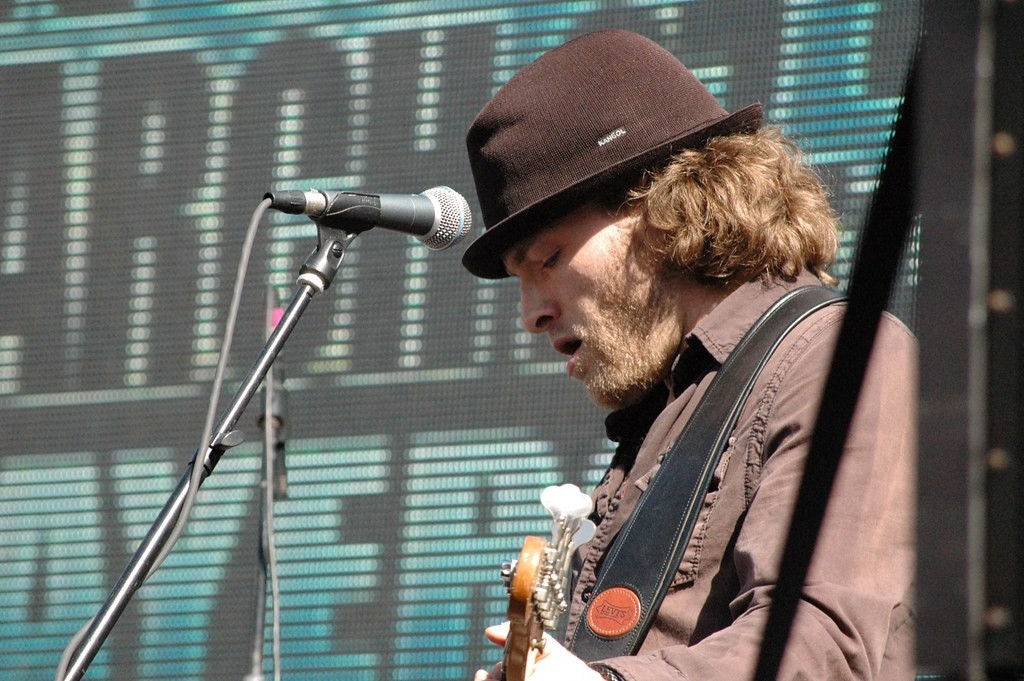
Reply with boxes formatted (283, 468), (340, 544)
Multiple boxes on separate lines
(462, 28), (925, 681)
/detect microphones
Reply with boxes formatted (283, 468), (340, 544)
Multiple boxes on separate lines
(263, 186), (473, 251)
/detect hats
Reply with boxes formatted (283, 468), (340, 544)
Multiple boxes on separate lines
(461, 29), (764, 279)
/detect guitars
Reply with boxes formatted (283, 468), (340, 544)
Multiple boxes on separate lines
(502, 481), (597, 680)
